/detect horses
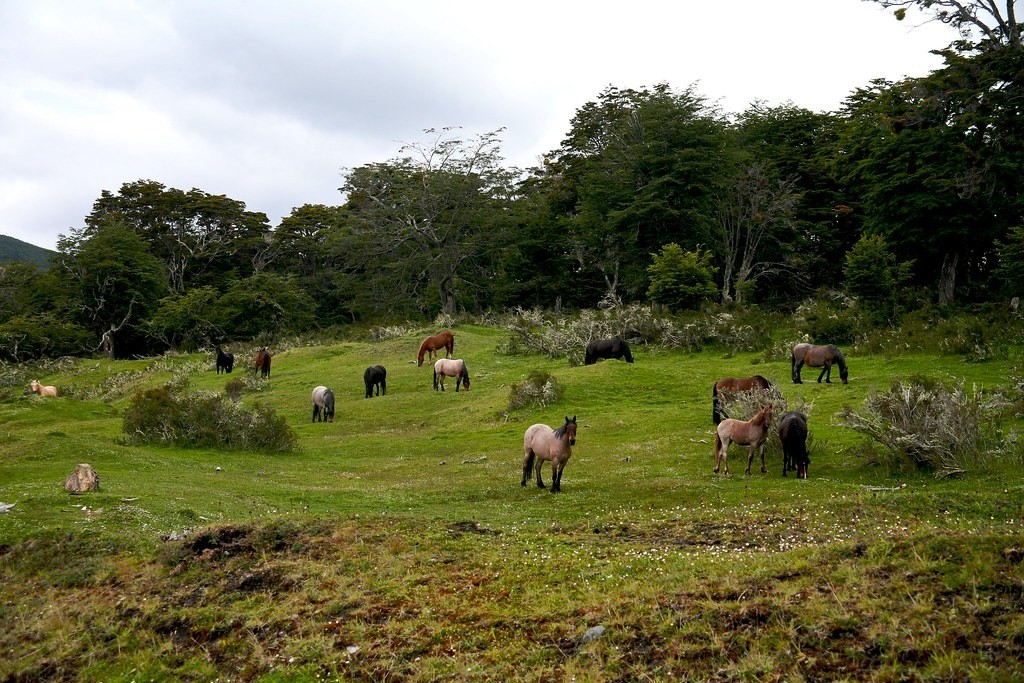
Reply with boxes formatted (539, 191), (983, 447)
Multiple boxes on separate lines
(30, 379), (59, 398)
(712, 375), (773, 426)
(585, 338), (634, 366)
(778, 410), (811, 481)
(364, 364), (387, 399)
(433, 358), (470, 392)
(417, 331), (454, 367)
(255, 347), (271, 380)
(521, 415), (577, 494)
(214, 344), (234, 375)
(791, 342), (849, 384)
(311, 385), (335, 423)
(713, 402), (773, 476)
